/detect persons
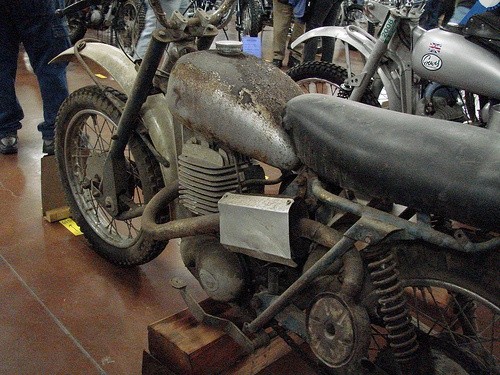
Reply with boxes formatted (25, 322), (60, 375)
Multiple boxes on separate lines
(0, 0), (89, 155)
(272, 0), (308, 69)
(135, 0), (190, 76)
(305, 0), (342, 83)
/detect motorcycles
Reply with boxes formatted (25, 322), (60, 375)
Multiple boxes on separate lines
(47, 0), (500, 375)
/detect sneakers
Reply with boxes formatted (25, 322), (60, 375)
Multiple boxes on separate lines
(0, 133), (19, 154)
(273, 59), (283, 68)
(42, 133), (90, 154)
(288, 57), (299, 67)
